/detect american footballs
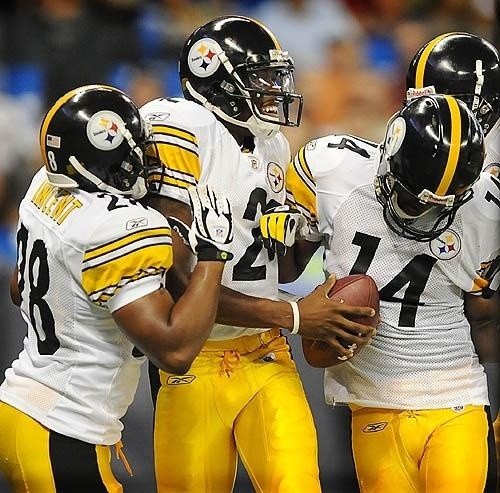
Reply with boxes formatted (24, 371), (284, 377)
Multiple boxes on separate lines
(301, 273), (379, 367)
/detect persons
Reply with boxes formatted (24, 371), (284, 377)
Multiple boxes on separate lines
(259, 90), (499, 492)
(404, 26), (499, 182)
(129, 12), (380, 493)
(0, 81), (235, 490)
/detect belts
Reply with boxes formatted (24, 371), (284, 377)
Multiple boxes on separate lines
(200, 326), (281, 351)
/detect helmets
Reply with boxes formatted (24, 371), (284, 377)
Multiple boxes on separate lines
(40, 85), (165, 199)
(406, 31), (500, 137)
(177, 16), (302, 137)
(374, 94), (484, 243)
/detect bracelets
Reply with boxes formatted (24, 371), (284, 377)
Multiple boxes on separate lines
(287, 300), (302, 335)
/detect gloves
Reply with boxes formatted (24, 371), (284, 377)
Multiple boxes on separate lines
(259, 206), (310, 261)
(167, 185), (237, 263)
